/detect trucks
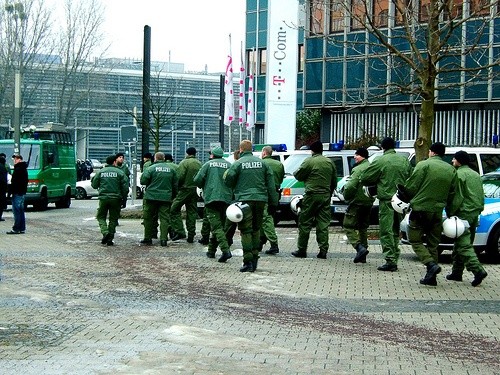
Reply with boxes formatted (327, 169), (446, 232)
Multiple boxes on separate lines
(0, 128), (76, 211)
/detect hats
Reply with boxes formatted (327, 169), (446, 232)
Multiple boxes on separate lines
(166, 154), (173, 162)
(310, 142), (323, 154)
(12, 154), (22, 160)
(187, 147), (196, 156)
(453, 151), (469, 165)
(211, 146), (224, 157)
(144, 153), (152, 158)
(382, 137), (395, 149)
(430, 142), (444, 158)
(115, 153), (124, 159)
(355, 148), (369, 158)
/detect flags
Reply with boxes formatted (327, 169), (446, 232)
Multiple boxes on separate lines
(246, 68), (255, 132)
(224, 55), (235, 126)
(239, 66), (246, 125)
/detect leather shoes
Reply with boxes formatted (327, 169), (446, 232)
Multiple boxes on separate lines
(353, 244), (368, 264)
(471, 270), (487, 286)
(317, 249), (328, 258)
(446, 273), (462, 281)
(291, 247), (306, 257)
(141, 227), (279, 272)
(420, 262), (442, 285)
(378, 263), (397, 271)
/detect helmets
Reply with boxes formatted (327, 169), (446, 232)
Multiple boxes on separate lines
(442, 216), (465, 238)
(226, 202), (249, 222)
(390, 183), (412, 214)
(290, 195), (304, 215)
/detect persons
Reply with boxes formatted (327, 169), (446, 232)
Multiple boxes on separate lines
(359, 137), (413, 271)
(140, 151), (178, 246)
(338, 149), (376, 263)
(0, 153), (12, 221)
(223, 139), (280, 272)
(446, 151), (488, 287)
(396, 142), (458, 285)
(7, 154), (29, 234)
(193, 147), (240, 263)
(90, 155), (128, 247)
(259, 145), (285, 255)
(114, 153), (131, 208)
(293, 140), (338, 258)
(76, 158), (94, 181)
(171, 148), (202, 243)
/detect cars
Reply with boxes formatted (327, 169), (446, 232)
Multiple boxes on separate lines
(279, 140), (382, 205)
(75, 174), (145, 199)
(399, 168), (500, 262)
(329, 139), (500, 224)
(195, 143), (295, 218)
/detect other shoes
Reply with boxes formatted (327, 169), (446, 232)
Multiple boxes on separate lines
(6, 229), (25, 234)
(101, 234), (114, 246)
